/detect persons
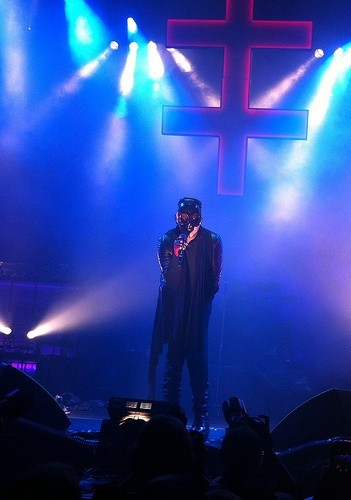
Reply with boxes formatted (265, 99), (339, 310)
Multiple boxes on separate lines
(154, 196), (225, 440)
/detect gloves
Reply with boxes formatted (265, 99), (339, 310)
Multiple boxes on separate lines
(173, 239), (187, 258)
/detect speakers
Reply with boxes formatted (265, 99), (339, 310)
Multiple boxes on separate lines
(0, 364), (72, 432)
(269, 387), (351, 450)
(107, 397), (188, 426)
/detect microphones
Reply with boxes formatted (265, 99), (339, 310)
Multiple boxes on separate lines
(182, 228), (189, 244)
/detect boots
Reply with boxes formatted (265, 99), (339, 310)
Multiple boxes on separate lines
(189, 391), (211, 440)
